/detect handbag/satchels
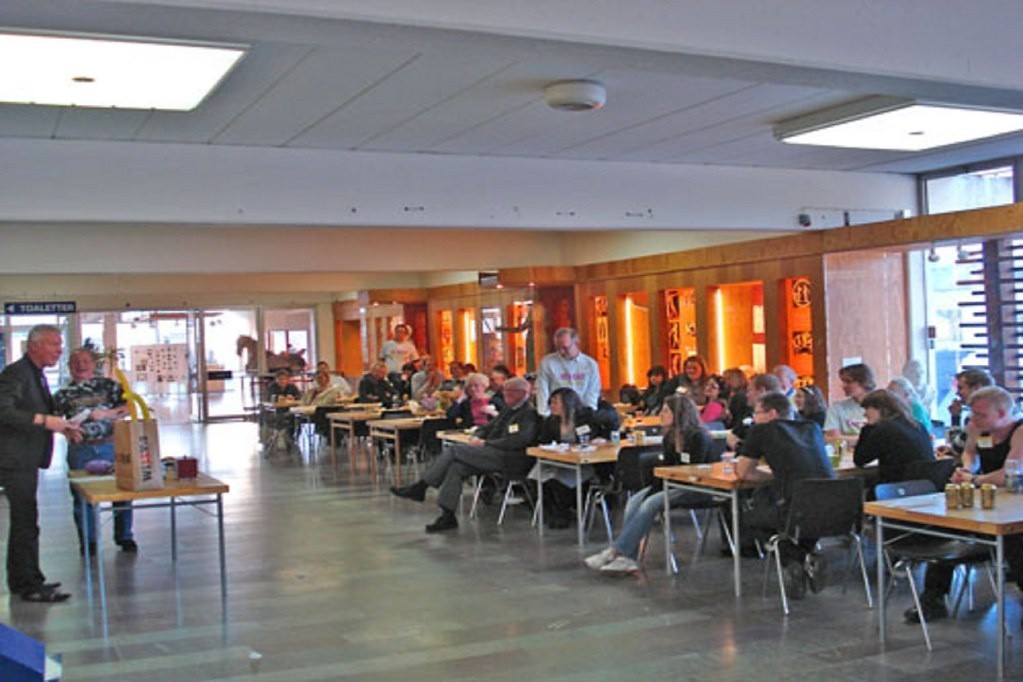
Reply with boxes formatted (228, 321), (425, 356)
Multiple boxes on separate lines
(114, 416), (164, 490)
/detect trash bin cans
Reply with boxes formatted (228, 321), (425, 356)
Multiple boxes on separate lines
(0, 622), (63, 682)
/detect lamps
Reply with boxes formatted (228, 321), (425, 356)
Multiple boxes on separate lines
(0, 27), (252, 113)
(773, 95), (1023, 152)
(541, 83), (605, 115)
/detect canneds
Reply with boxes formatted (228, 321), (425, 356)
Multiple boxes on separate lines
(944, 482), (974, 509)
(980, 483), (997, 509)
(636, 430), (646, 446)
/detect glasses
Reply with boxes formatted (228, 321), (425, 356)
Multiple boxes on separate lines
(752, 411), (762, 416)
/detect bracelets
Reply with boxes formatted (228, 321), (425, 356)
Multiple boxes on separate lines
(42, 414), (47, 427)
(970, 475), (980, 482)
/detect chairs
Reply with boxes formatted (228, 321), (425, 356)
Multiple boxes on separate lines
(249, 363), (1023, 682)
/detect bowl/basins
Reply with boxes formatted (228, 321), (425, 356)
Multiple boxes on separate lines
(827, 454), (843, 468)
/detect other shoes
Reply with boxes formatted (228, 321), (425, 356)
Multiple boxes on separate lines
(12, 582), (61, 594)
(116, 539), (136, 551)
(22, 586), (71, 602)
(787, 564), (805, 598)
(427, 516), (457, 530)
(81, 542), (96, 554)
(584, 549), (615, 569)
(600, 556), (639, 576)
(391, 486), (424, 502)
(905, 594), (946, 620)
(804, 552), (826, 592)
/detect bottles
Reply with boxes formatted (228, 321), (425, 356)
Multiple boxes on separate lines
(403, 394), (408, 407)
(85, 459), (115, 475)
(392, 394), (402, 409)
(636, 407), (643, 422)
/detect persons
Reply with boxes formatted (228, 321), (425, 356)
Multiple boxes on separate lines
(538, 388), (605, 529)
(52, 348), (138, 555)
(304, 361), (352, 448)
(536, 327), (600, 419)
(0, 325), (87, 600)
(823, 359), (1023, 623)
(382, 323), (419, 373)
(583, 355), (838, 597)
(389, 361), (539, 531)
(358, 354), (445, 460)
(266, 370), (301, 453)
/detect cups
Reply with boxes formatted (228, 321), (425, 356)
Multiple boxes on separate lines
(579, 434), (590, 447)
(722, 454), (736, 473)
(611, 431), (620, 444)
(179, 458), (197, 477)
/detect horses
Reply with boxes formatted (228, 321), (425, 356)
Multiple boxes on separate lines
(237, 334), (308, 403)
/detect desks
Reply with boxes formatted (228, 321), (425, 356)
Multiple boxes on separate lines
(436, 426), (499, 518)
(527, 428), (734, 549)
(364, 408), (449, 486)
(325, 408), (414, 464)
(863, 483), (1023, 682)
(290, 403), (382, 453)
(261, 395), (360, 450)
(654, 450), (955, 598)
(69, 459), (229, 642)
